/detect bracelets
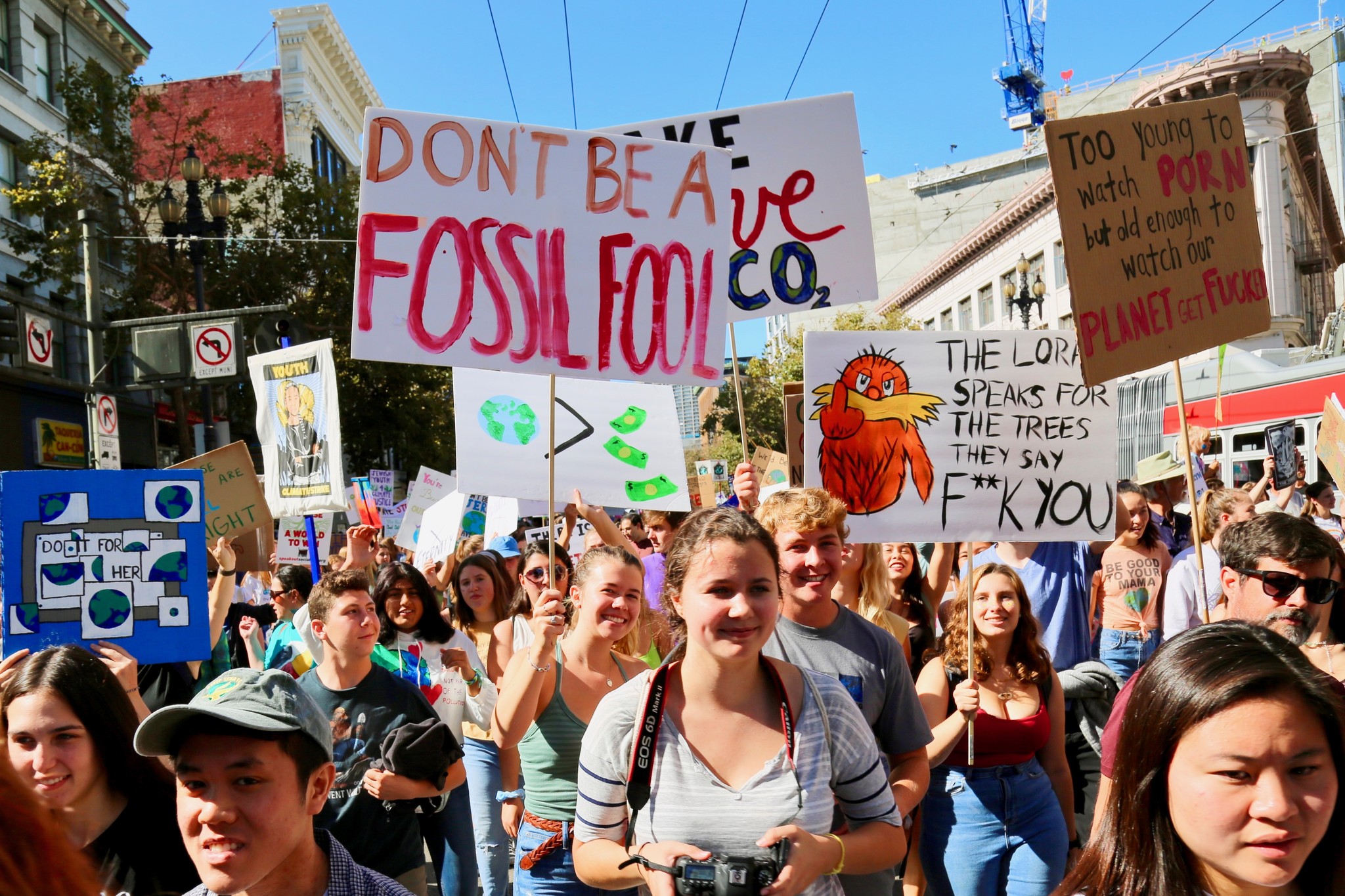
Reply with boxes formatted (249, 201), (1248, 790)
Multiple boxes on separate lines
(496, 788), (526, 804)
(219, 567), (236, 577)
(528, 650), (553, 673)
(463, 668), (484, 689)
(824, 832), (846, 879)
(125, 687), (139, 693)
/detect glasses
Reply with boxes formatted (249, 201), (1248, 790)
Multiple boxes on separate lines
(1298, 464), (1306, 471)
(1231, 567), (1343, 604)
(269, 589), (292, 600)
(207, 570), (218, 577)
(523, 566), (569, 585)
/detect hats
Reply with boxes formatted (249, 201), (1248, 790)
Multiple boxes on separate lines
(489, 536), (521, 558)
(1132, 450), (1195, 486)
(133, 667), (333, 762)
(1300, 456), (1308, 462)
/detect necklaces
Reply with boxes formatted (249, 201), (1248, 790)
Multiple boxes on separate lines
(1304, 640), (1335, 675)
(998, 691), (1013, 701)
(579, 654), (614, 688)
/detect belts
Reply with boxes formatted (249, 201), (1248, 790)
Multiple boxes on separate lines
(518, 809), (574, 871)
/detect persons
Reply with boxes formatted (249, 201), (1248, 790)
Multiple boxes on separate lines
(0, 424), (1345, 896)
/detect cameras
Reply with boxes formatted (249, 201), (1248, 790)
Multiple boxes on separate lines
(672, 838), (789, 896)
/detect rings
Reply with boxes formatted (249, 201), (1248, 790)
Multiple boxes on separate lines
(543, 604), (553, 615)
(549, 615), (557, 626)
